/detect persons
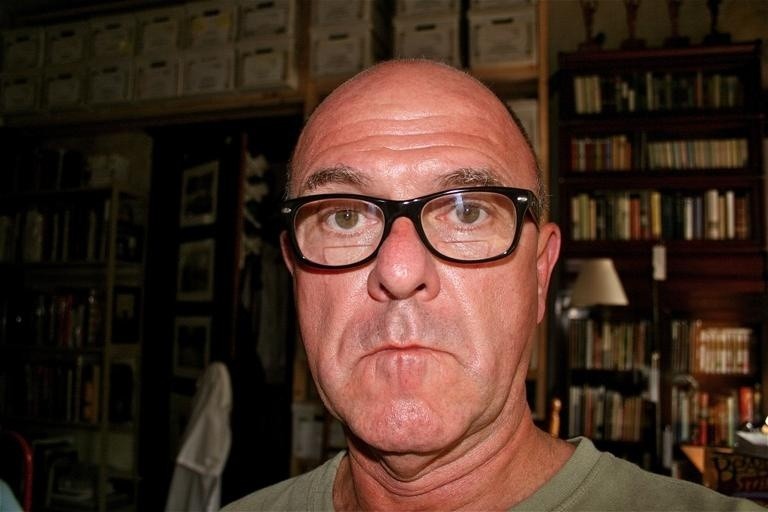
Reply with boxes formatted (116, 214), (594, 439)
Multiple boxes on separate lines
(207, 56), (768, 512)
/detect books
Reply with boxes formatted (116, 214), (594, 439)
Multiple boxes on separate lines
(670, 320), (762, 449)
(0, 130), (111, 431)
(570, 68), (752, 243)
(566, 319), (645, 442)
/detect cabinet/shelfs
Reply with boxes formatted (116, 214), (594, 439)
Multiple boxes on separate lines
(296, 324), (353, 477)
(149, 117), (295, 512)
(3, 180), (139, 511)
(546, 55), (764, 484)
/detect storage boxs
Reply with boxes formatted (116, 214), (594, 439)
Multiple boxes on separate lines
(133, 6), (186, 101)
(1, 26), (45, 115)
(467, 0), (539, 65)
(391, 0), (463, 72)
(90, 13), (134, 107)
(308, 0), (378, 83)
(46, 19), (90, 112)
(237, 2), (304, 91)
(186, 0), (236, 96)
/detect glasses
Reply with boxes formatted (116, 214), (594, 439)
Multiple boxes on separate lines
(278, 186), (542, 271)
